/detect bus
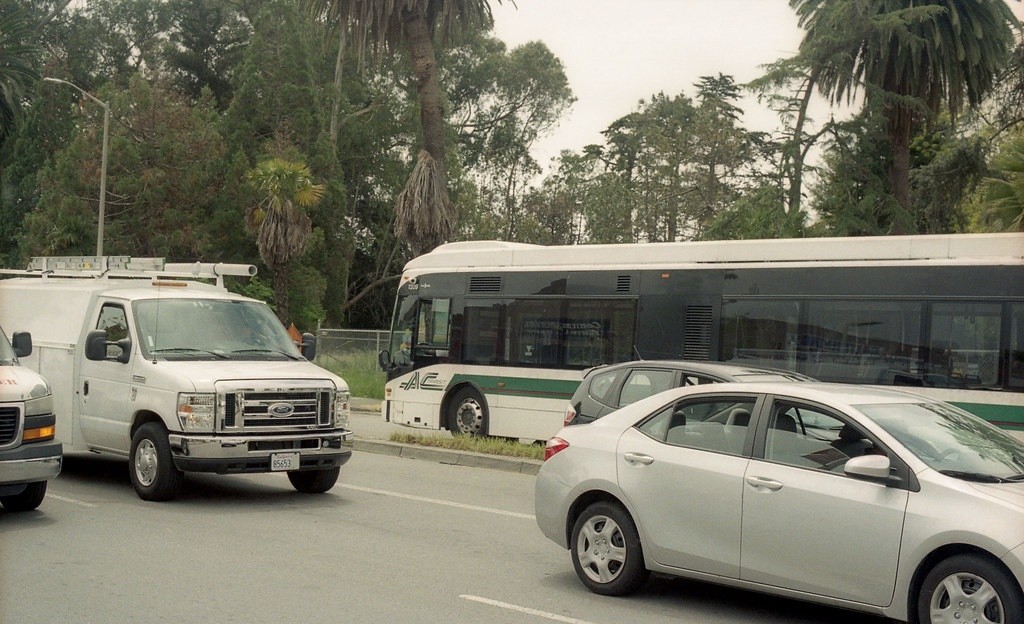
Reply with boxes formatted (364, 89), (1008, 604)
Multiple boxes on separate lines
(377, 230), (1023, 450)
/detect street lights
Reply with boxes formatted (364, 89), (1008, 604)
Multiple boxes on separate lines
(42, 75), (110, 269)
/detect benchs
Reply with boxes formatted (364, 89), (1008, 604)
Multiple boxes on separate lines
(655, 408), (752, 458)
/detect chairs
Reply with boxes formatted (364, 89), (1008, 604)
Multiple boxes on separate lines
(827, 423), (874, 459)
(767, 414), (798, 463)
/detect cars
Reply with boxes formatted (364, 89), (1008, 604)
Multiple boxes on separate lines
(564, 359), (849, 444)
(536, 380), (1024, 624)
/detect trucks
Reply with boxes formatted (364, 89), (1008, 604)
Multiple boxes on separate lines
(0, 254), (354, 503)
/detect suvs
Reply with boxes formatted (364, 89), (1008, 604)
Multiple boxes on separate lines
(0, 325), (64, 511)
